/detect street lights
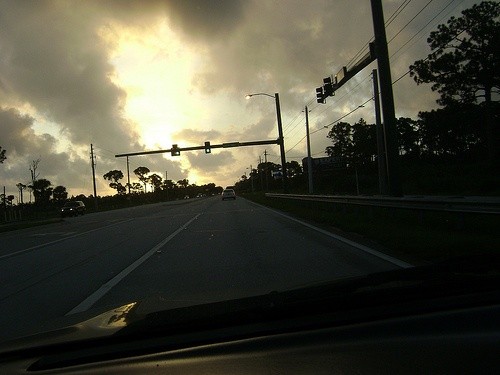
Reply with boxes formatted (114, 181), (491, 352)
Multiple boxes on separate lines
(245, 92), (288, 192)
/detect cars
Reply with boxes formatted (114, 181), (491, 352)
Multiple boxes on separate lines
(183, 192), (221, 200)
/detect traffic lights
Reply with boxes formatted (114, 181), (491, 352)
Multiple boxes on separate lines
(205, 141), (211, 154)
(315, 86), (324, 104)
(172, 144), (180, 156)
(323, 77), (333, 97)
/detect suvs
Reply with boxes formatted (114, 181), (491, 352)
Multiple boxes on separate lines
(59, 201), (86, 218)
(221, 189), (236, 200)
